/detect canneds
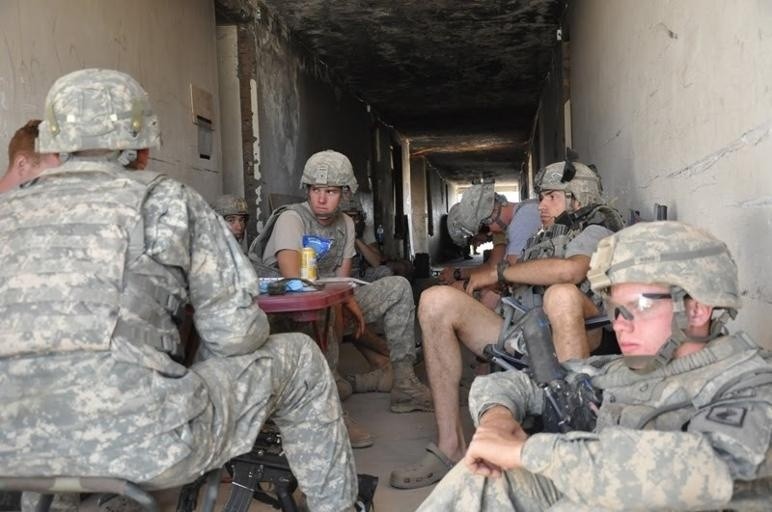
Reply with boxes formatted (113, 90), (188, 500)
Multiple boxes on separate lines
(300, 248), (317, 282)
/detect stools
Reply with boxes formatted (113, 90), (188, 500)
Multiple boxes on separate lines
(1, 458), (222, 510)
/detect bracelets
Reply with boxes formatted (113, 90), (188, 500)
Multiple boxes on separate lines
(496, 258), (510, 285)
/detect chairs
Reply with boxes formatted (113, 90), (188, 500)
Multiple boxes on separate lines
(482, 202), (669, 379)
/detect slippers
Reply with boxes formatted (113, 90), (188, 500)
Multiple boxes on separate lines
(389, 441), (456, 490)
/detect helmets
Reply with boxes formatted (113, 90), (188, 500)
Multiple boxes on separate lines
(33, 67), (164, 155)
(533, 160), (602, 208)
(586, 220), (744, 311)
(209, 193), (251, 220)
(342, 194), (363, 211)
(298, 148), (359, 196)
(458, 182), (495, 236)
(446, 201), (473, 248)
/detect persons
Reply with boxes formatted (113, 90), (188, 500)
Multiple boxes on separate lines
(425, 202), (508, 314)
(410, 219), (771, 510)
(0, 117), (63, 197)
(389, 160), (636, 491)
(0, 67), (372, 511)
(246, 146), (433, 450)
(332, 197), (393, 285)
(209, 193), (394, 400)
(455, 182), (549, 407)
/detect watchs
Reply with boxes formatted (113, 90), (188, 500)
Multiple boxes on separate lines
(453, 268), (462, 282)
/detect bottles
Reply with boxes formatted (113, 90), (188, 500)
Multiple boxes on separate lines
(377, 224), (384, 243)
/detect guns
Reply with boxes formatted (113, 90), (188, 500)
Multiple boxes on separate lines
(498, 296), (611, 352)
(479, 308), (600, 433)
(175, 427), (378, 512)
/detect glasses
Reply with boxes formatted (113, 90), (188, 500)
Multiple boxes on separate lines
(603, 292), (691, 323)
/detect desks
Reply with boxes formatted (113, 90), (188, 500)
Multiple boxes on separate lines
(176, 278), (357, 356)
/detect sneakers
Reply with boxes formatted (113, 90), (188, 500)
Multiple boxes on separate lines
(336, 378), (353, 401)
(422, 379), (470, 406)
(390, 376), (436, 414)
(371, 360), (392, 392)
(342, 406), (374, 448)
